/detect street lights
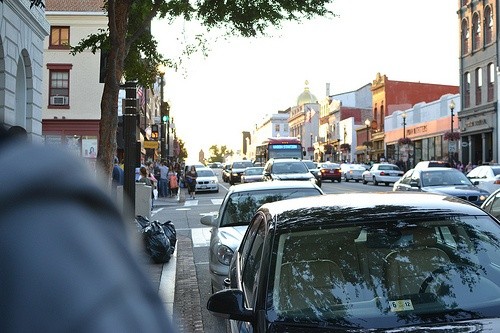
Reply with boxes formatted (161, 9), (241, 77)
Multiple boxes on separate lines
(401, 111), (408, 170)
(448, 99), (456, 167)
(310, 131), (312, 160)
(364, 118), (370, 165)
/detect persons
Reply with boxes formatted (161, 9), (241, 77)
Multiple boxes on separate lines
(443, 156), (472, 173)
(138, 158), (181, 199)
(186, 165), (198, 200)
(112, 156), (124, 187)
(382, 157), (407, 173)
(0, 124), (180, 333)
(184, 165), (189, 188)
(340, 158), (376, 167)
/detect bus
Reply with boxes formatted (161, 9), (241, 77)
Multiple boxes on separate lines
(255, 137), (306, 167)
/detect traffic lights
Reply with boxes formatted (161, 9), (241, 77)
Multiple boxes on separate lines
(161, 102), (169, 122)
(152, 124), (159, 139)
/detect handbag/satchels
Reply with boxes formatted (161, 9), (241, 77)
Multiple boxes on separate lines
(186, 177), (193, 183)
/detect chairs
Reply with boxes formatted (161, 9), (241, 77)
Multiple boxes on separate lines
(279, 242), (345, 310)
(235, 197), (257, 222)
(385, 226), (452, 296)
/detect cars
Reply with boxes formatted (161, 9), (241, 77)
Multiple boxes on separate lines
(480, 188), (500, 223)
(200, 180), (325, 294)
(466, 165), (500, 196)
(195, 168), (219, 194)
(206, 192), (500, 333)
(318, 163), (342, 183)
(393, 166), (492, 207)
(362, 163), (405, 186)
(239, 166), (264, 184)
(222, 164), (231, 182)
(184, 162), (205, 173)
(340, 163), (366, 182)
(207, 162), (226, 168)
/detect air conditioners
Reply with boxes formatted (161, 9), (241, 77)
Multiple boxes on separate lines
(52, 96), (64, 105)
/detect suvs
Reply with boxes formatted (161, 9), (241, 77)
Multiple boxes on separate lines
(228, 160), (254, 185)
(415, 160), (450, 168)
(261, 157), (319, 186)
(302, 160), (322, 187)
